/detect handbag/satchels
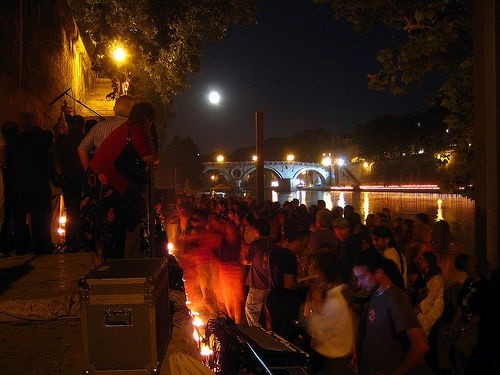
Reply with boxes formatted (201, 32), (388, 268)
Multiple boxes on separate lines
(114, 143), (146, 184)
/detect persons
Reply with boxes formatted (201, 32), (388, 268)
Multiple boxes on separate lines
(149, 184), (461, 326)
(351, 259), (432, 375)
(300, 258), (350, 375)
(259, 247), (329, 374)
(77, 94), (137, 262)
(463, 266), (500, 375)
(0, 114), (97, 255)
(435, 258), (495, 375)
(411, 253), (444, 338)
(87, 104), (158, 261)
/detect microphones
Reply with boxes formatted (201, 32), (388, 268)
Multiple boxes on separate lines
(49, 86), (71, 106)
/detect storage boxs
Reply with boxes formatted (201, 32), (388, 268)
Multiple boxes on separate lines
(78, 256), (176, 375)
